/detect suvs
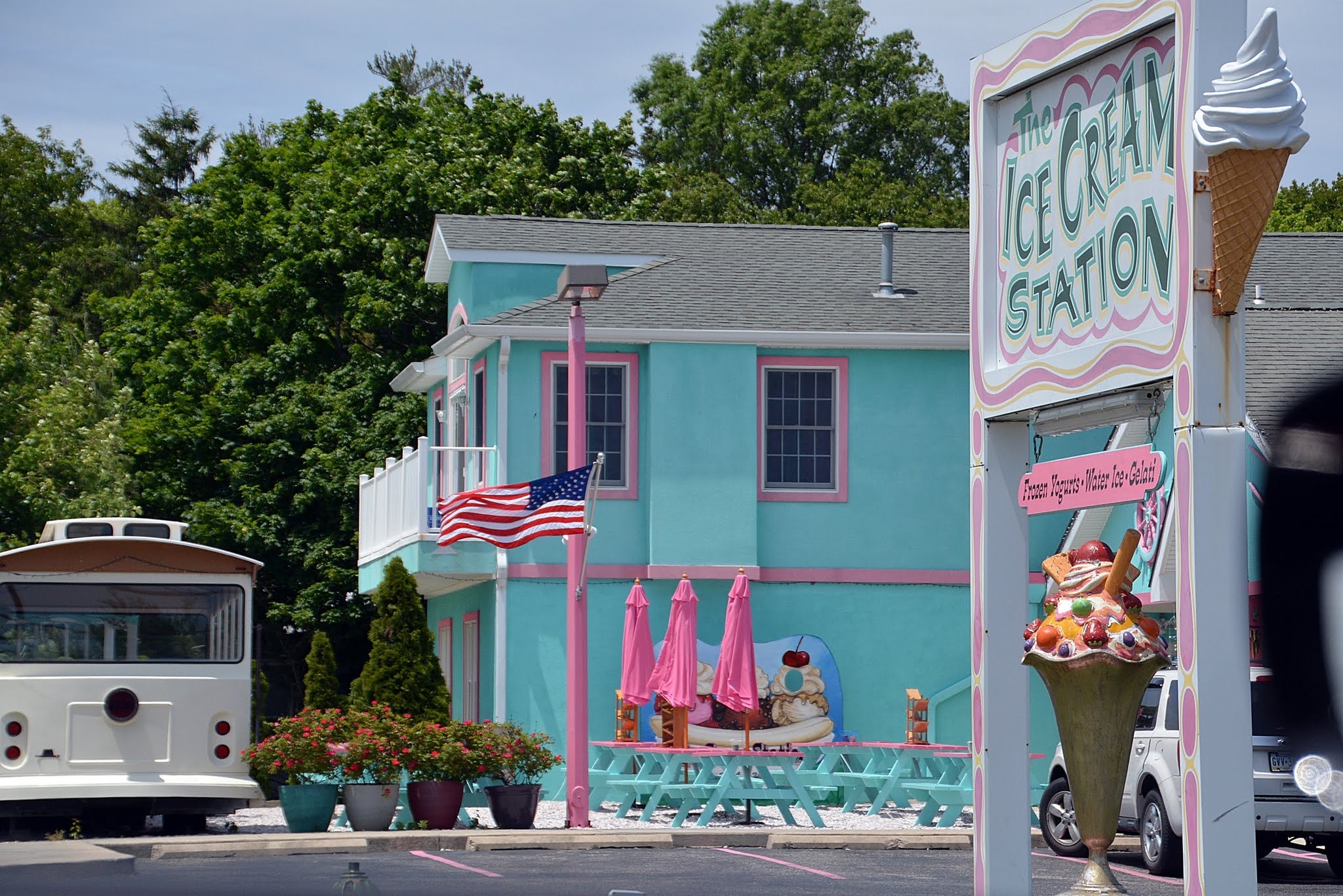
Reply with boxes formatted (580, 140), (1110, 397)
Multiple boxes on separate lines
(1037, 667), (1342, 881)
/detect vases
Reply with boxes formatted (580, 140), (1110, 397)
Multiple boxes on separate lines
(405, 780), (463, 829)
(342, 783), (400, 832)
(484, 783), (544, 829)
(277, 784), (340, 832)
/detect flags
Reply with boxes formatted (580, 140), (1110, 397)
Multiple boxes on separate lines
(433, 461), (597, 550)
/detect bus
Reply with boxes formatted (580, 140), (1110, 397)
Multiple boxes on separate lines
(0, 515), (265, 846)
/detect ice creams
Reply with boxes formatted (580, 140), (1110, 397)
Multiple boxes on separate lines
(1190, 6), (1308, 314)
(1018, 529), (1170, 896)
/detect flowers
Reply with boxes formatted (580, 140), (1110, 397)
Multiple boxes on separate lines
(238, 700), (567, 786)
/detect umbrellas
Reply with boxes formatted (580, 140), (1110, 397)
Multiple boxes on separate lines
(622, 576), (654, 808)
(645, 568), (699, 785)
(711, 568), (761, 822)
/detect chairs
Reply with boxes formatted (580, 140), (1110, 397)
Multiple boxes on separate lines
(588, 731), (1049, 839)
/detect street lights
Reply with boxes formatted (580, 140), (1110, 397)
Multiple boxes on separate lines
(554, 263), (612, 827)
